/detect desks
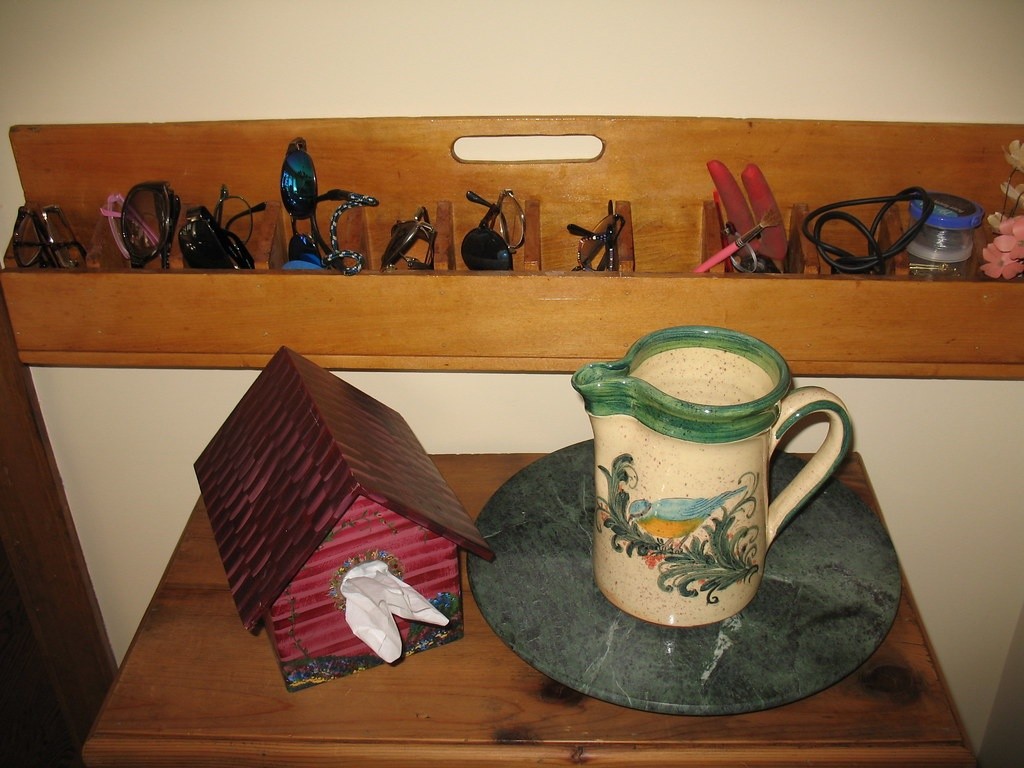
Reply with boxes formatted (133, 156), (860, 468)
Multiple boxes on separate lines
(79, 450), (977, 768)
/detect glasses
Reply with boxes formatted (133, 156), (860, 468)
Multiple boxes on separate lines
(100, 181), (181, 271)
(722, 222), (757, 273)
(461, 189), (526, 271)
(567, 200), (625, 272)
(379, 206), (436, 273)
(12, 201), (87, 268)
(280, 136), (348, 273)
(179, 185), (268, 269)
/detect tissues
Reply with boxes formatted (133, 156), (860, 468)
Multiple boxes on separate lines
(192, 344), (496, 692)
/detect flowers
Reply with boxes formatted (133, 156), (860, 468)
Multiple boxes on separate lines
(979, 140), (1024, 281)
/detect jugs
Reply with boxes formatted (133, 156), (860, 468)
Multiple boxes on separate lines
(570, 326), (851, 626)
